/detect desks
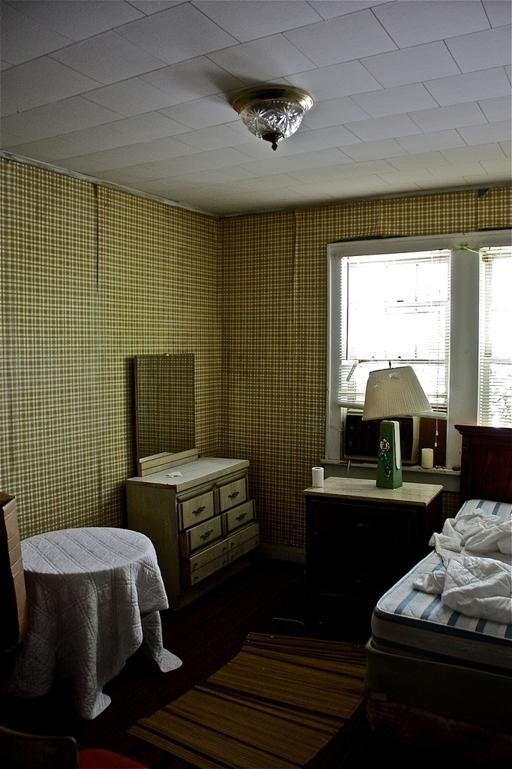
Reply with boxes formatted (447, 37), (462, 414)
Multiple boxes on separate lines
(20, 525), (182, 720)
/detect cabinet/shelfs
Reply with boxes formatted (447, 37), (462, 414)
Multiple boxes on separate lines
(122, 457), (261, 611)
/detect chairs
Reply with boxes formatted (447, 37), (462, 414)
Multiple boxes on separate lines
(1, 726), (149, 768)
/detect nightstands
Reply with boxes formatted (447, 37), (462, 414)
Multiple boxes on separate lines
(302, 475), (444, 626)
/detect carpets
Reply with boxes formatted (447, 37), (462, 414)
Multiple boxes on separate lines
(130, 633), (369, 768)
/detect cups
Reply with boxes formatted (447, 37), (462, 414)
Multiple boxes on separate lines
(421, 448), (434, 469)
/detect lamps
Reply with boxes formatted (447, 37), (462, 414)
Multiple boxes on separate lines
(361, 356), (433, 488)
(229, 79), (313, 152)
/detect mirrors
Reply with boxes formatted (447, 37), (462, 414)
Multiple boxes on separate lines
(131, 350), (195, 478)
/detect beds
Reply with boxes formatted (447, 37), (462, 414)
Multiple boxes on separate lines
(360, 419), (511, 769)
